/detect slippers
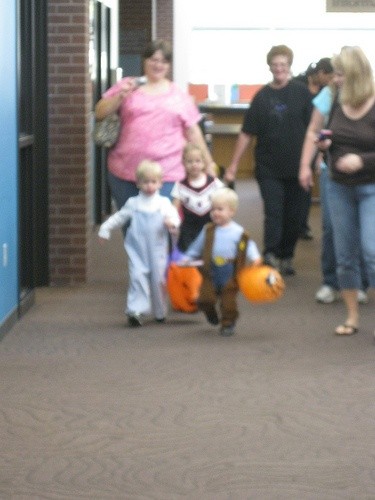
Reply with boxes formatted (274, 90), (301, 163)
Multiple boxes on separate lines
(336, 322), (359, 335)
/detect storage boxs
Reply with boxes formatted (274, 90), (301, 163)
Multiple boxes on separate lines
(208, 82), (239, 103)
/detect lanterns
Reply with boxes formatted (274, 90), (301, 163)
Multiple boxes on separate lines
(163, 250), (203, 312)
(238, 264), (286, 302)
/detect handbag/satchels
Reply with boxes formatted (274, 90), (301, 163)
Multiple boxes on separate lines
(92, 78), (132, 149)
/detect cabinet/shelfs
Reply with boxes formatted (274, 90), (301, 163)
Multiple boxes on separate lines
(200, 101), (256, 177)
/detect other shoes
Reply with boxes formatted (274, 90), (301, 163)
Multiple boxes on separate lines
(128, 315), (141, 327)
(156, 318), (165, 321)
(264, 252), (279, 272)
(206, 308), (218, 326)
(358, 289), (368, 302)
(222, 324), (233, 336)
(280, 259), (295, 274)
(316, 285), (341, 304)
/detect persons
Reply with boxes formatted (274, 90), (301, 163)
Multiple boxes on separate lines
(223, 46), (313, 274)
(170, 141), (225, 250)
(93, 40), (216, 236)
(294, 57), (335, 240)
(98, 161), (181, 329)
(299, 46), (375, 335)
(183, 188), (261, 337)
